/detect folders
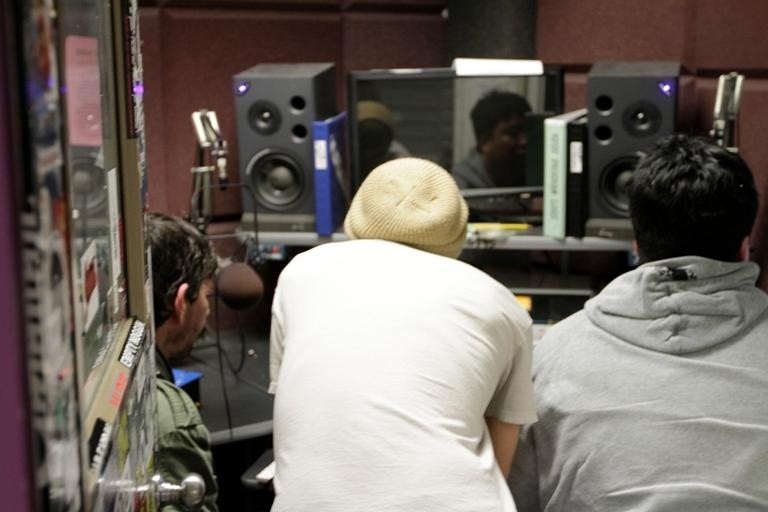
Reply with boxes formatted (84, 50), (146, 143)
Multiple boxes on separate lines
(313, 111), (346, 236)
(564, 115), (587, 238)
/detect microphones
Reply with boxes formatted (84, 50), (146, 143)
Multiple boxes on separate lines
(210, 256), (265, 308)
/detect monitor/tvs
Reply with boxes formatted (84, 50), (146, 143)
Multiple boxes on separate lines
(345, 63), (561, 210)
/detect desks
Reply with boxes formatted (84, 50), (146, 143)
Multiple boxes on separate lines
(235, 224), (640, 323)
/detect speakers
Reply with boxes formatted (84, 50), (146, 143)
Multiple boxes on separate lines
(585, 56), (684, 239)
(231, 60), (338, 230)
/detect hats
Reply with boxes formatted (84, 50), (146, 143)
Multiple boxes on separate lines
(342, 156), (470, 259)
(356, 101), (395, 134)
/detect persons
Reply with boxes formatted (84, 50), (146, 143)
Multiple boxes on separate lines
(509, 133), (768, 512)
(142, 208), (226, 511)
(448, 88), (533, 191)
(358, 97), (409, 189)
(265, 157), (537, 511)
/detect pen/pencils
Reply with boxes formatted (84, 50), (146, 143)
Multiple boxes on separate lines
(468, 223), (532, 230)
